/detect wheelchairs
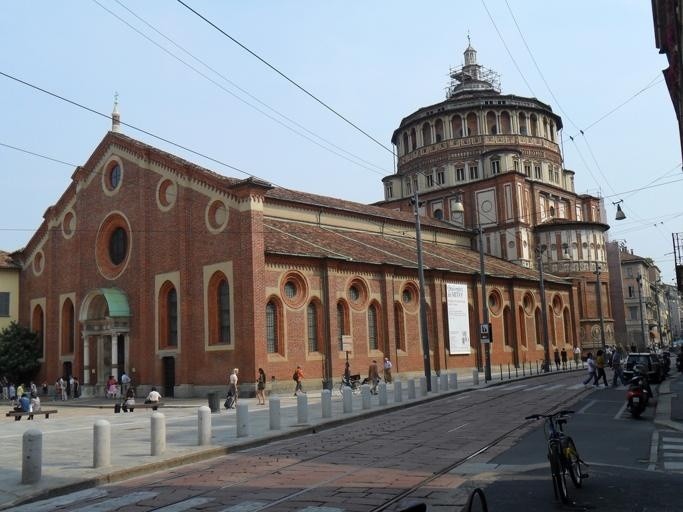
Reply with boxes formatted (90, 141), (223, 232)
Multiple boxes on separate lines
(340, 375), (363, 396)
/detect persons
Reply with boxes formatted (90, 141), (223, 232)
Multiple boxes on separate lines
(554, 343), (638, 388)
(255, 368), (266, 405)
(369, 360), (381, 395)
(107, 373), (135, 412)
(2, 375), (79, 420)
(229, 368), (240, 408)
(145, 387), (162, 411)
(293, 367), (306, 395)
(384, 358), (392, 384)
(343, 362), (351, 385)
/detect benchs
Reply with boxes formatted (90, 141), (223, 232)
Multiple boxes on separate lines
(6, 409), (60, 420)
(122, 403), (163, 412)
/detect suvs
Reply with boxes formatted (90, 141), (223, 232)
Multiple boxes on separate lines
(623, 352), (665, 382)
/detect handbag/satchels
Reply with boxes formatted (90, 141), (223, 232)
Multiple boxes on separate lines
(293, 374), (298, 381)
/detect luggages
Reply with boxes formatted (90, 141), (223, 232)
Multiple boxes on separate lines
(224, 392), (238, 409)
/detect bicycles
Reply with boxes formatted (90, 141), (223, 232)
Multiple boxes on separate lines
(526, 410), (590, 501)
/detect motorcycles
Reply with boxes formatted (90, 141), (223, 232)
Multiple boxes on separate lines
(659, 352), (671, 374)
(620, 366), (648, 419)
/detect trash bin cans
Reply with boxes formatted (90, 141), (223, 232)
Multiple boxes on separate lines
(670, 382), (683, 421)
(208, 391), (220, 413)
